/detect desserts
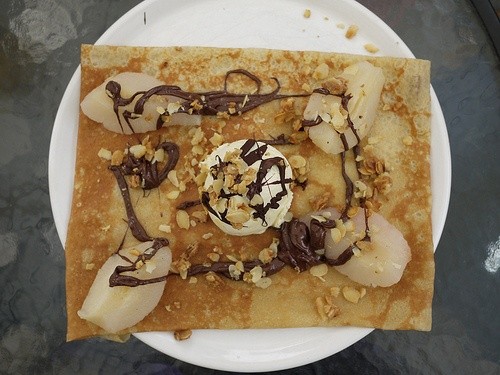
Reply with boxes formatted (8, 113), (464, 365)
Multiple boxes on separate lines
(196, 139), (294, 237)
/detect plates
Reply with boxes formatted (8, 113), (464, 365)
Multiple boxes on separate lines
(44, 1), (454, 373)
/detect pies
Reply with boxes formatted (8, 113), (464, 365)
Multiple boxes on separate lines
(65, 43), (436, 344)
